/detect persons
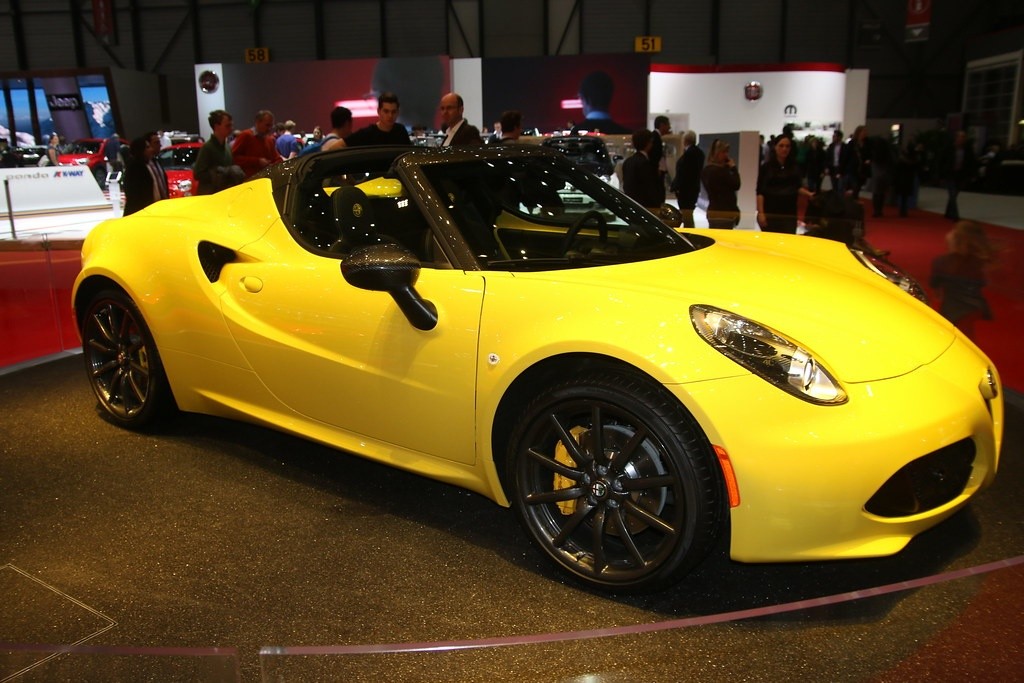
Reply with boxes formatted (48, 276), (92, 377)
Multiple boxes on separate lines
(326, 91), (414, 151)
(45, 132), (61, 168)
(646, 115), (671, 179)
(439, 92), (483, 147)
(621, 129), (666, 208)
(567, 119), (579, 137)
(192, 109), (246, 196)
(0, 137), (25, 168)
(702, 138), (741, 230)
(321, 107), (352, 152)
(927, 220), (994, 325)
(577, 70), (633, 135)
(102, 133), (120, 173)
(500, 109), (523, 144)
(122, 128), (174, 216)
(193, 91), (503, 213)
(755, 134), (817, 233)
(755, 125), (1006, 245)
(670, 130), (705, 228)
(230, 109), (283, 179)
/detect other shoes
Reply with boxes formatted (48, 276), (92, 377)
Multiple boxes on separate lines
(943, 214), (959, 222)
(842, 200), (908, 218)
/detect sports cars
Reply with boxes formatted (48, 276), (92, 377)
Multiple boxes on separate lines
(70, 146), (1006, 589)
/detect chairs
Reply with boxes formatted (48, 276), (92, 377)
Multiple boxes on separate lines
(423, 179), (489, 262)
(331, 186), (399, 252)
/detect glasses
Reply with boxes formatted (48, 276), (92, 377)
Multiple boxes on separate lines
(439, 105), (459, 110)
(150, 140), (160, 145)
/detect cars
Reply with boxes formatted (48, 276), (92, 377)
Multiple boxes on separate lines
(155, 140), (204, 197)
(57, 136), (133, 185)
(540, 135), (624, 208)
